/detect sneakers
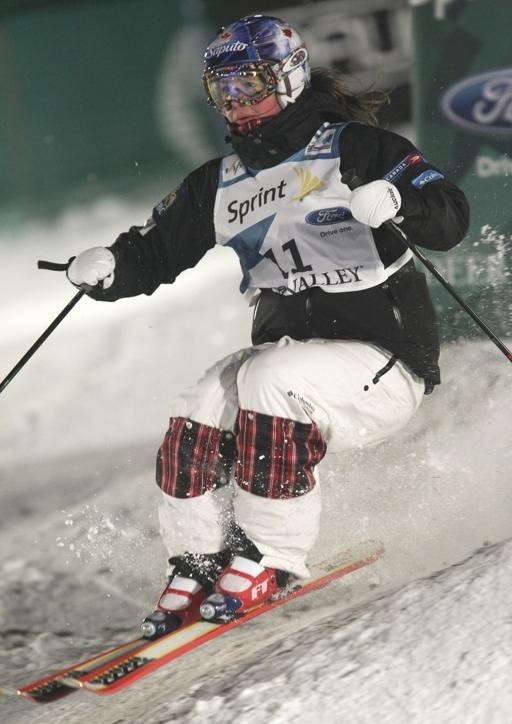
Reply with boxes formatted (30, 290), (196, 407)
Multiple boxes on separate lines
(219, 546), (289, 593)
(159, 550), (231, 611)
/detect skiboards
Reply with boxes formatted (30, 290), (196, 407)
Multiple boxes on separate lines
(5, 537), (385, 706)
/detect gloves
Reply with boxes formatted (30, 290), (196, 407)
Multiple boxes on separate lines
(67, 247), (116, 286)
(349, 180), (401, 228)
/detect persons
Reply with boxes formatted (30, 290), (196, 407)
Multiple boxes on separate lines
(66, 13), (470, 625)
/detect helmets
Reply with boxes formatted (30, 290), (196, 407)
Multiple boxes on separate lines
(201, 14), (311, 136)
